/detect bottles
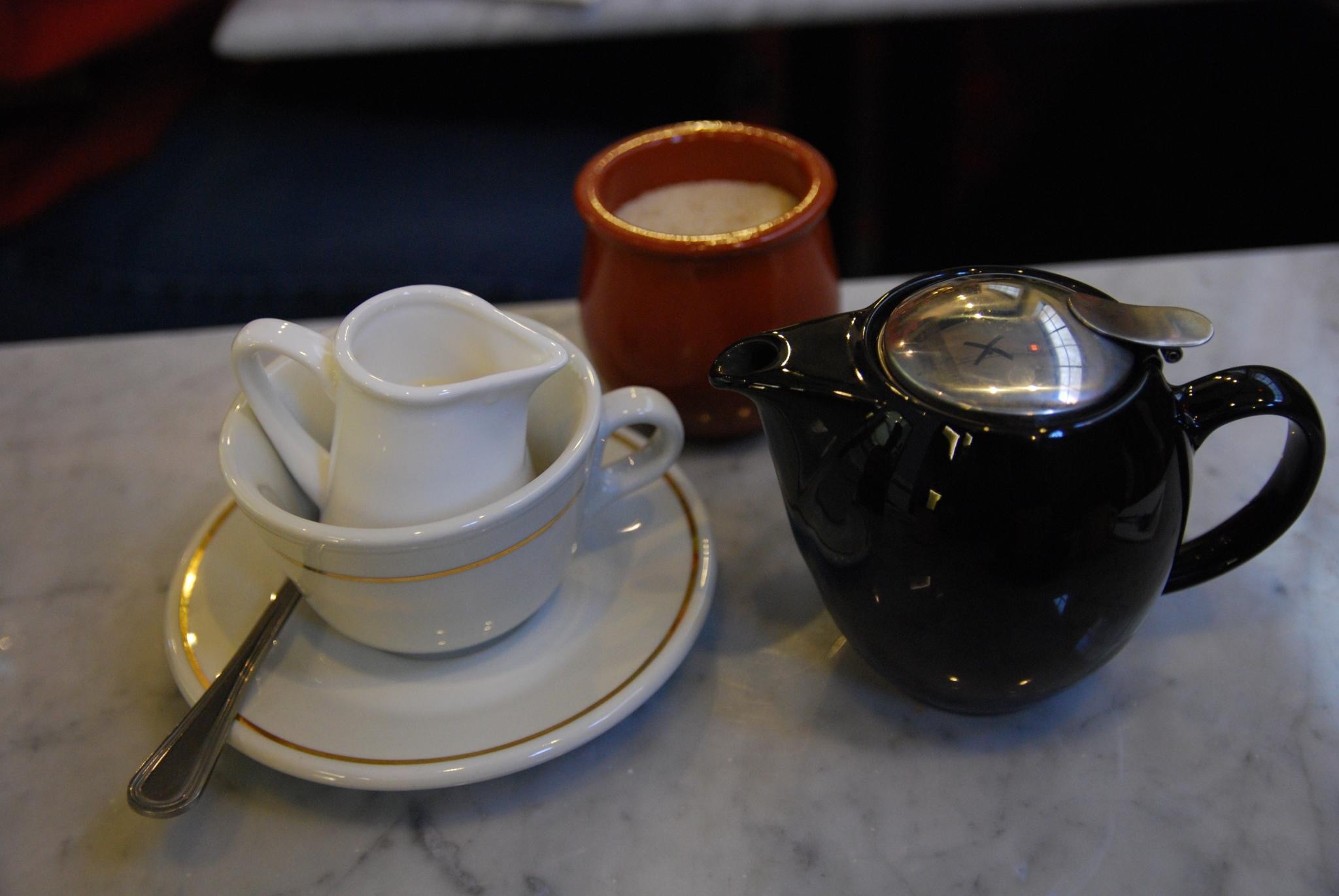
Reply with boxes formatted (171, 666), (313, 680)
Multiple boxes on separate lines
(572, 120), (843, 446)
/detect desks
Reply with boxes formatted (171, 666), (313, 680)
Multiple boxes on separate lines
(1, 244), (1339, 896)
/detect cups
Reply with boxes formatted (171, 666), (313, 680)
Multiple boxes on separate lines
(217, 312), (686, 654)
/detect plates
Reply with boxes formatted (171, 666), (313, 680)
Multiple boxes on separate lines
(162, 428), (716, 791)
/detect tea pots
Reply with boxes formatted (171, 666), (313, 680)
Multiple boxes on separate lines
(706, 265), (1327, 719)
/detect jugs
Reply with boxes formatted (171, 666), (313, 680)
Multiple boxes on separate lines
(231, 284), (568, 529)
